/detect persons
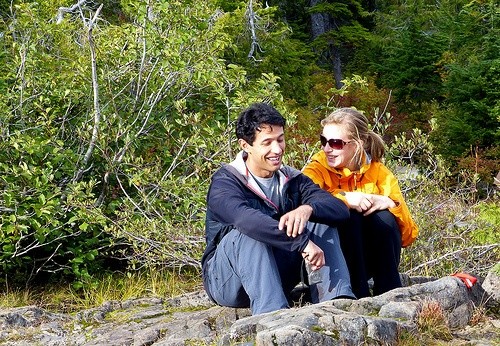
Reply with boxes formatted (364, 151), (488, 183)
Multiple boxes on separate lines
(301, 107), (418, 300)
(201, 102), (357, 316)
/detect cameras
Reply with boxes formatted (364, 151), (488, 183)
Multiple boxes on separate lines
(305, 262), (323, 286)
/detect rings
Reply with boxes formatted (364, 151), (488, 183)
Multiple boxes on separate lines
(295, 218), (300, 222)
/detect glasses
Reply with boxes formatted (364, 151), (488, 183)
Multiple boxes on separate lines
(320, 135), (352, 150)
